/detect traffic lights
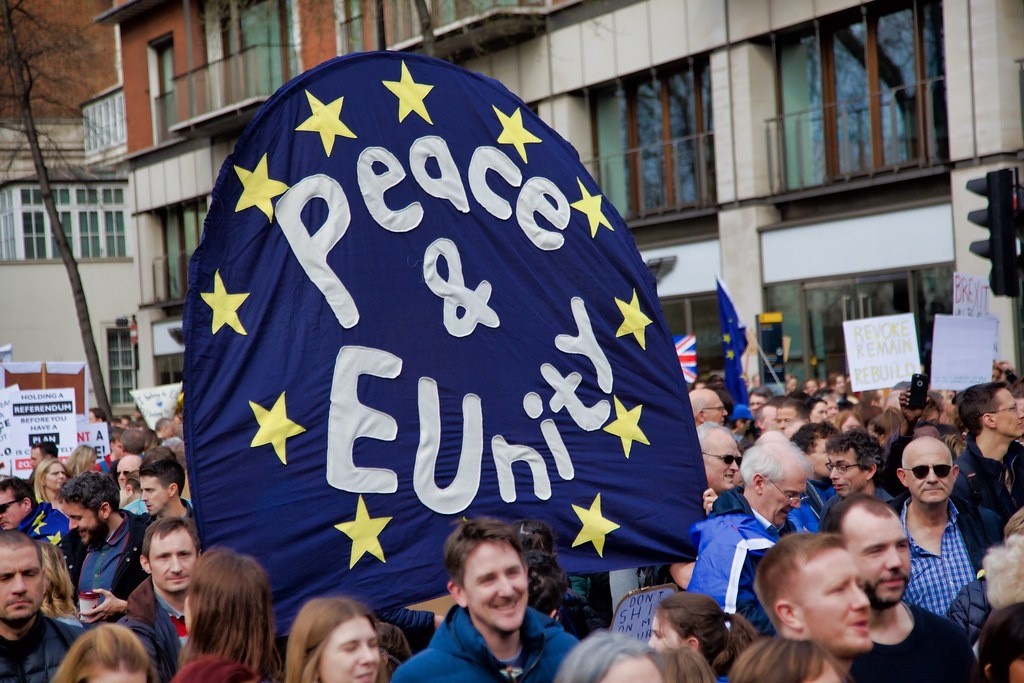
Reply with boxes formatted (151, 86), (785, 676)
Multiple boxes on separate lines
(964, 169), (1019, 299)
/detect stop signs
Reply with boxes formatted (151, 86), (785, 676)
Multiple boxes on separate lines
(130, 322), (137, 344)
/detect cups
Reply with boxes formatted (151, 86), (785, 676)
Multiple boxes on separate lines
(77, 591), (100, 620)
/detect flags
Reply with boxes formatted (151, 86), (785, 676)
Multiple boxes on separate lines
(715, 279), (750, 406)
(670, 333), (699, 385)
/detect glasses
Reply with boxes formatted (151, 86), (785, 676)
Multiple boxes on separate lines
(0, 498), (22, 514)
(702, 406), (725, 411)
(975, 406), (1018, 419)
(903, 465), (952, 479)
(826, 462), (861, 473)
(702, 452), (742, 466)
(766, 476), (809, 504)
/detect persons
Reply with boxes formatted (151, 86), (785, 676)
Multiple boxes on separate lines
(0, 361), (1024, 683)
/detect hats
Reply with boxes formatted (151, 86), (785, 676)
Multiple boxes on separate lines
(730, 404), (754, 420)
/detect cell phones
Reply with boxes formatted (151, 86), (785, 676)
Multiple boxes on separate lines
(908, 373), (929, 410)
